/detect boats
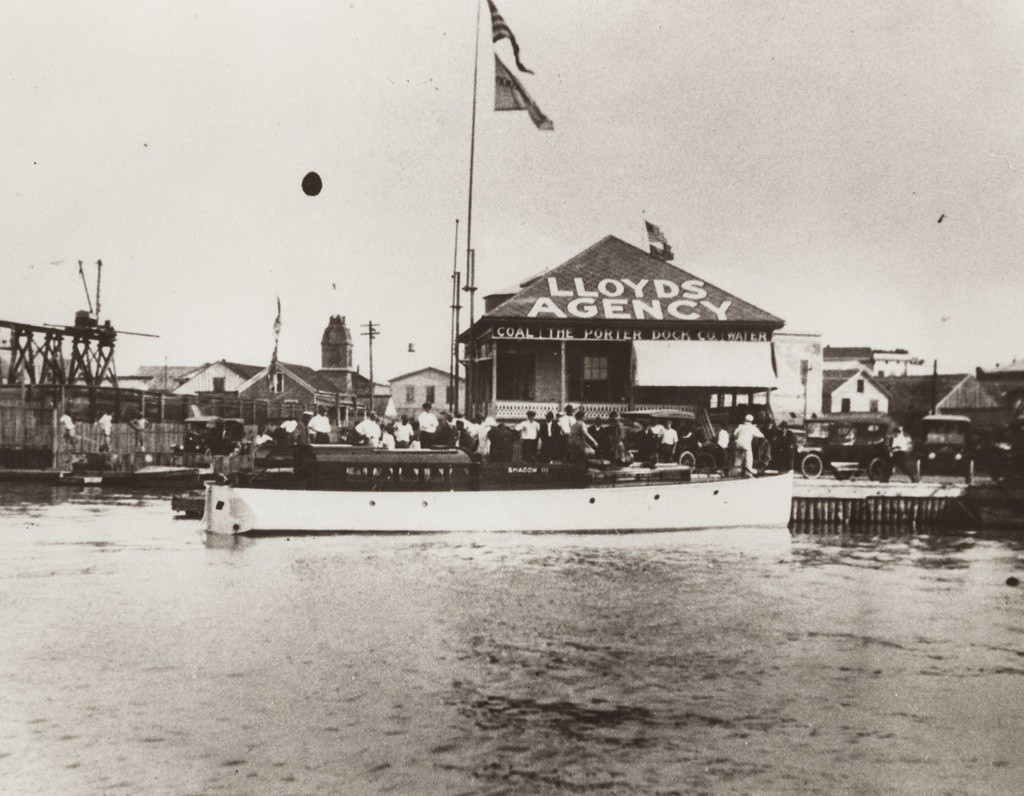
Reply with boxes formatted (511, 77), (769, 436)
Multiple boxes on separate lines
(134, 465), (228, 489)
(201, 441), (794, 536)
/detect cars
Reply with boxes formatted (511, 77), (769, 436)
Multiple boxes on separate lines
(170, 416), (251, 456)
(791, 414), (896, 483)
(909, 415), (975, 483)
(622, 410), (712, 473)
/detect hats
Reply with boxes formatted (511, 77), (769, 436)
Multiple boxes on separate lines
(302, 411), (313, 417)
(564, 405), (577, 413)
(779, 421), (790, 428)
(526, 411), (536, 415)
(745, 414), (754, 422)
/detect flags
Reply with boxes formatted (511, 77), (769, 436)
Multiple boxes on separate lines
(650, 243), (674, 262)
(494, 52), (555, 131)
(487, 0), (535, 75)
(646, 221), (668, 245)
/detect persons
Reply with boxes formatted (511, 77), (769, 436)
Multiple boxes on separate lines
(60, 402), (798, 478)
